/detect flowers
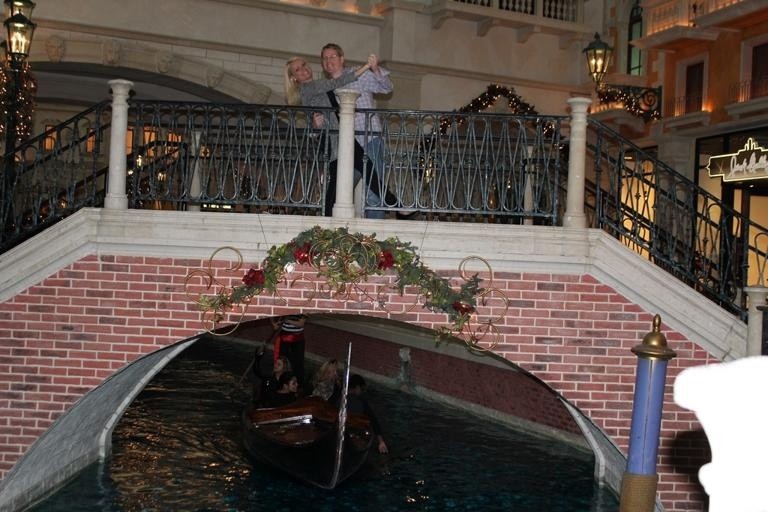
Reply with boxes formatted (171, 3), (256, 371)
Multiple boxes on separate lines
(193, 224), (494, 353)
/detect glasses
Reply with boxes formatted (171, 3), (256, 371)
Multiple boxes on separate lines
(323, 55), (339, 60)
(360, 385), (366, 392)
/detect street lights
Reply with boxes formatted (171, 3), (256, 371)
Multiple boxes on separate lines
(0, 0), (40, 234)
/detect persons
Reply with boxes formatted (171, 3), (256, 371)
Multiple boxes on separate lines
(287, 56), (419, 219)
(311, 43), (394, 219)
(242, 316), (389, 456)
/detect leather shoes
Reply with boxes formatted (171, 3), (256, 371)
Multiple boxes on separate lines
(396, 210), (420, 220)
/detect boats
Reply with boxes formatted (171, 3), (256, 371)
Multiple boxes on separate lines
(237, 395), (379, 491)
